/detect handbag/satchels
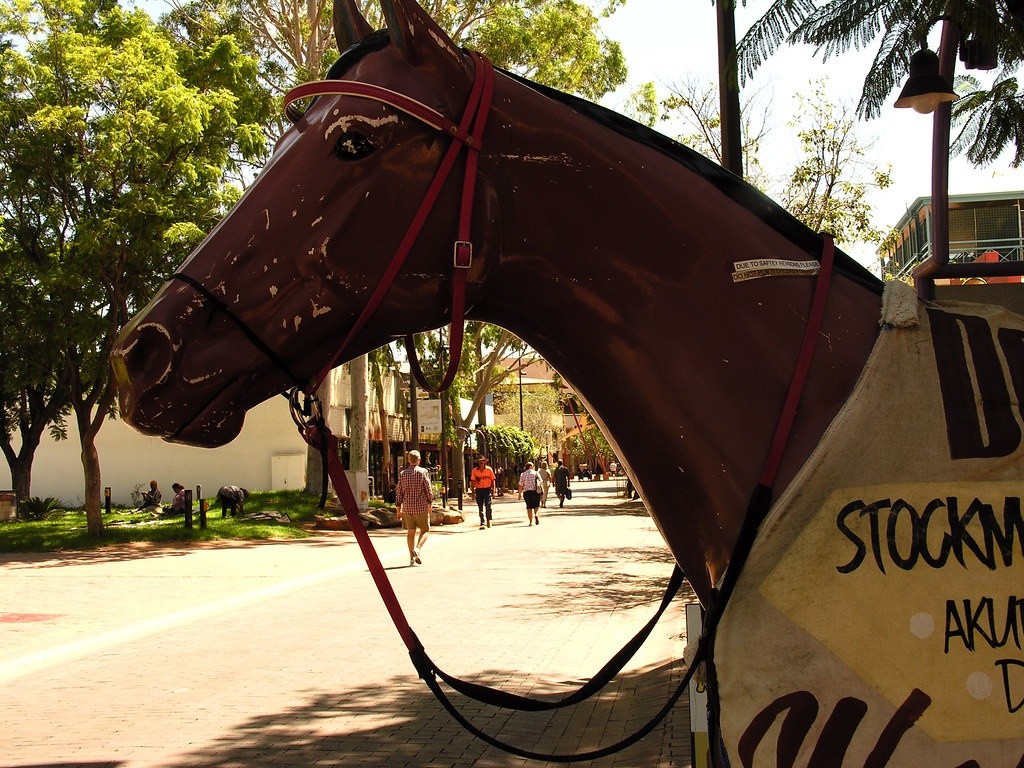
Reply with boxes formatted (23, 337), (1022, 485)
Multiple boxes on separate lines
(566, 486), (572, 500)
(535, 472), (542, 494)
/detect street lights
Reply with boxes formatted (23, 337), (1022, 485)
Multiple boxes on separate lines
(517, 370), (528, 432)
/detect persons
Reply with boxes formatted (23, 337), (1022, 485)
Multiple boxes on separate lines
(517, 462), (543, 526)
(117, 481), (161, 514)
(578, 467), (590, 481)
(470, 454), (496, 530)
(395, 450), (433, 565)
(218, 486), (249, 519)
(150, 483), (185, 519)
(538, 460), (571, 507)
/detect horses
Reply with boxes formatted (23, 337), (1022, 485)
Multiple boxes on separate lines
(111, 0), (1023, 767)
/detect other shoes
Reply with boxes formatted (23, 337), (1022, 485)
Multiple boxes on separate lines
(412, 547), (421, 564)
(535, 516), (539, 524)
(410, 560), (414, 566)
(479, 525), (484, 530)
(487, 522), (492, 528)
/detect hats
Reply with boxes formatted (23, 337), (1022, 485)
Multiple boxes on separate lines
(474, 455), (489, 464)
